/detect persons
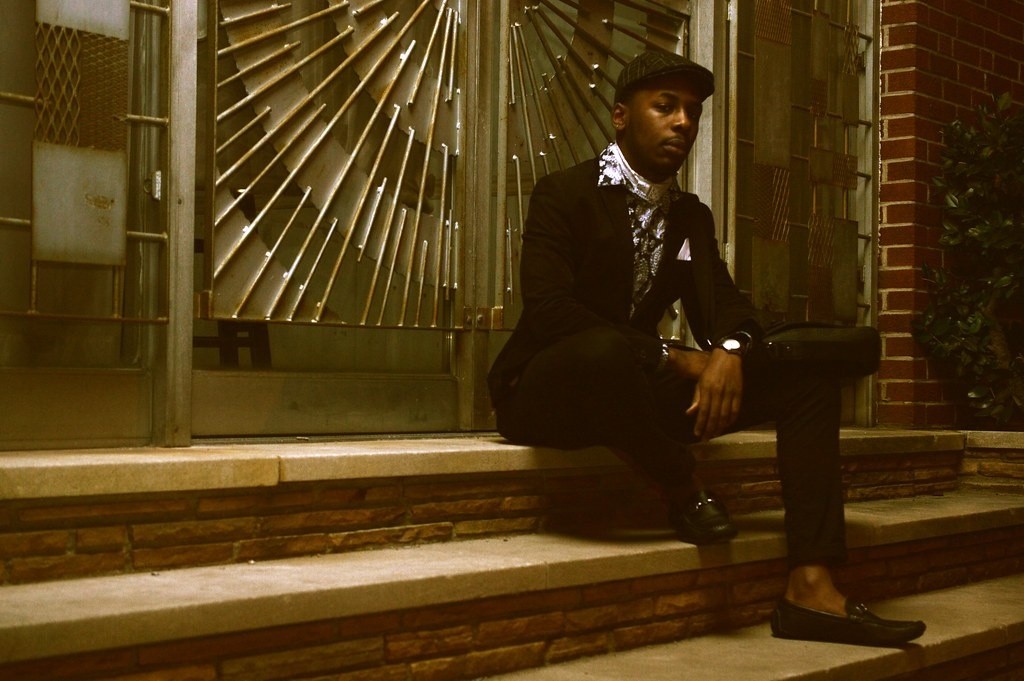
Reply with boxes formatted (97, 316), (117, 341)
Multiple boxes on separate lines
(486, 48), (926, 647)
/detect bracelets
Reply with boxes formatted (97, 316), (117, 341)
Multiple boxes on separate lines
(655, 344), (669, 373)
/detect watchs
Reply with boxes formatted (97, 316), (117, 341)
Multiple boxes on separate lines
(715, 339), (746, 359)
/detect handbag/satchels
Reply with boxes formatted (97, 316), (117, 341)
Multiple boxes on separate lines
(755, 321), (881, 377)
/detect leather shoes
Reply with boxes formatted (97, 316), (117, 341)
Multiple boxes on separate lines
(770, 598), (927, 645)
(671, 489), (738, 543)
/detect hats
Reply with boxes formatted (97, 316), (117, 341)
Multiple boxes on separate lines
(615, 51), (715, 103)
